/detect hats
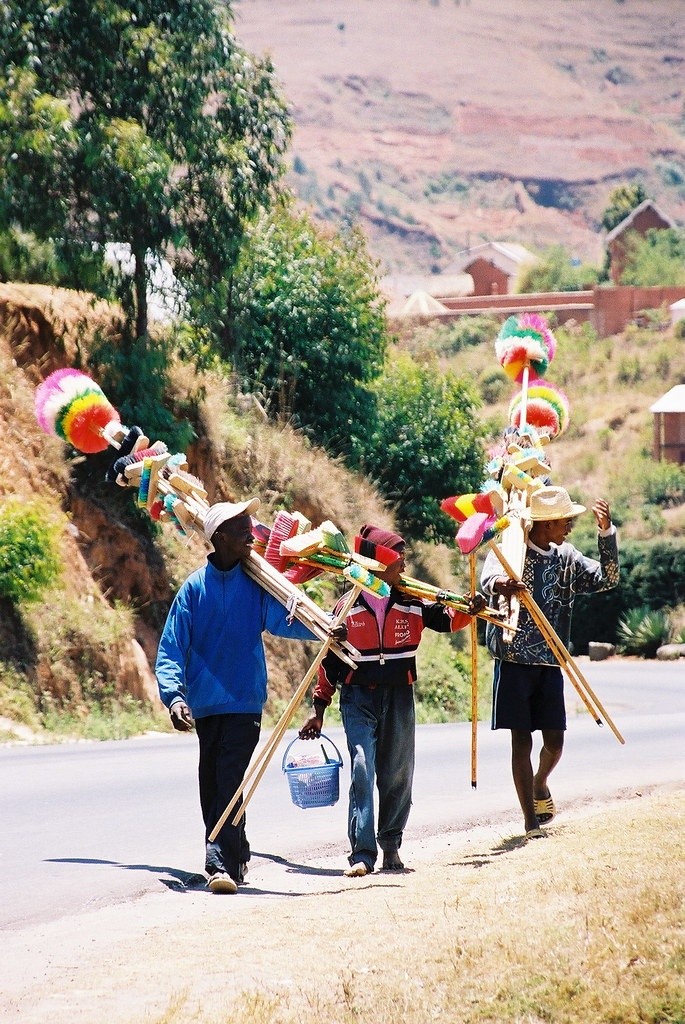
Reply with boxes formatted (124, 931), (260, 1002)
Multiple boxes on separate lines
(358, 523), (407, 548)
(521, 485), (587, 520)
(204, 498), (261, 541)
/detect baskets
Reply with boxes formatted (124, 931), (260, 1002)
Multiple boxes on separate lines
(282, 733), (344, 810)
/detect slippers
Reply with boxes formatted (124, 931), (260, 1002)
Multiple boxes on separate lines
(526, 828), (547, 841)
(533, 786), (555, 825)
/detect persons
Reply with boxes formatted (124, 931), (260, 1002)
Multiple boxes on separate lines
(480, 486), (619, 845)
(299, 525), (487, 876)
(154, 498), (348, 893)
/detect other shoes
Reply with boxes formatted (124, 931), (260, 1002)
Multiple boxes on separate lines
(209, 872), (236, 893)
(239, 862), (249, 880)
